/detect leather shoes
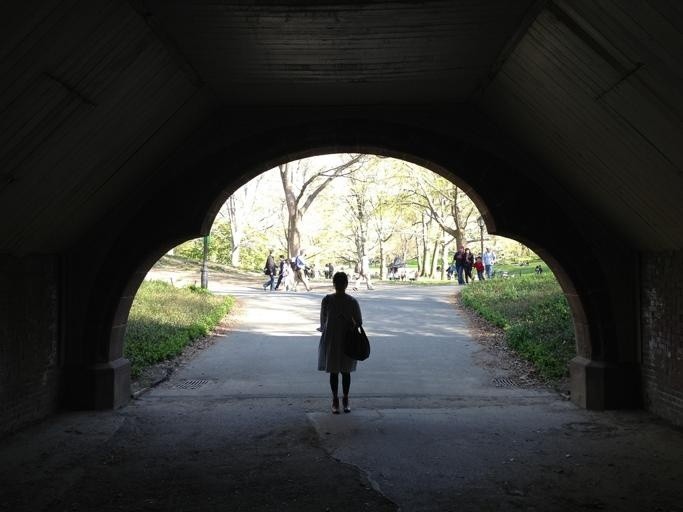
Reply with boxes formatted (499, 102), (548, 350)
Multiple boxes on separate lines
(342, 398), (351, 413)
(331, 402), (340, 414)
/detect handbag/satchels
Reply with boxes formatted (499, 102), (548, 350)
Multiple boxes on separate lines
(264, 269), (277, 276)
(345, 325), (370, 361)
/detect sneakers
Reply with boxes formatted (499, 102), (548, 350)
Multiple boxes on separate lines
(263, 285), (310, 292)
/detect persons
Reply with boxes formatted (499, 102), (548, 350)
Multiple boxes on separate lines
(446, 245), (497, 285)
(323, 264), (329, 279)
(315, 271), (363, 414)
(325, 263), (333, 279)
(262, 248), (314, 293)
(352, 255), (376, 292)
